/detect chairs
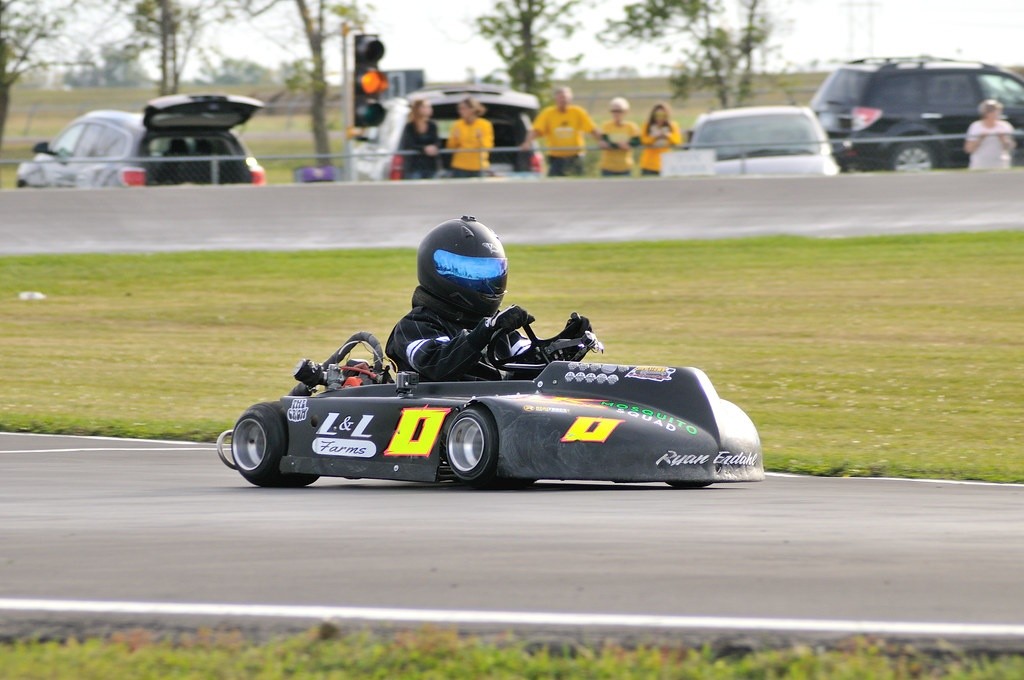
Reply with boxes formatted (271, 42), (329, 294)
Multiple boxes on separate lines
(163, 139), (188, 156)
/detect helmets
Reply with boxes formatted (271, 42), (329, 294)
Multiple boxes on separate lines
(418, 219), (508, 318)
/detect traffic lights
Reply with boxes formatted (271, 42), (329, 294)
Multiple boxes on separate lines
(354, 34), (386, 128)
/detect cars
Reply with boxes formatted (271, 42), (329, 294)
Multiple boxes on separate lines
(688, 105), (840, 177)
(350, 83), (547, 181)
(16, 93), (265, 189)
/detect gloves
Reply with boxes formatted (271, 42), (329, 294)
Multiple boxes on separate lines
(567, 312), (593, 338)
(489, 305), (533, 329)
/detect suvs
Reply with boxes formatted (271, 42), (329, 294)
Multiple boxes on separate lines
(811, 55), (1024, 171)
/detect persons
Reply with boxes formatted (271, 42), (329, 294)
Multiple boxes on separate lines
(447, 97), (494, 178)
(639, 103), (681, 176)
(386, 215), (593, 383)
(400, 100), (438, 179)
(600, 98), (641, 176)
(965, 100), (1016, 169)
(521, 87), (608, 177)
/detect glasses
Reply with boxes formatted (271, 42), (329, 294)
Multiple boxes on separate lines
(611, 109), (622, 113)
